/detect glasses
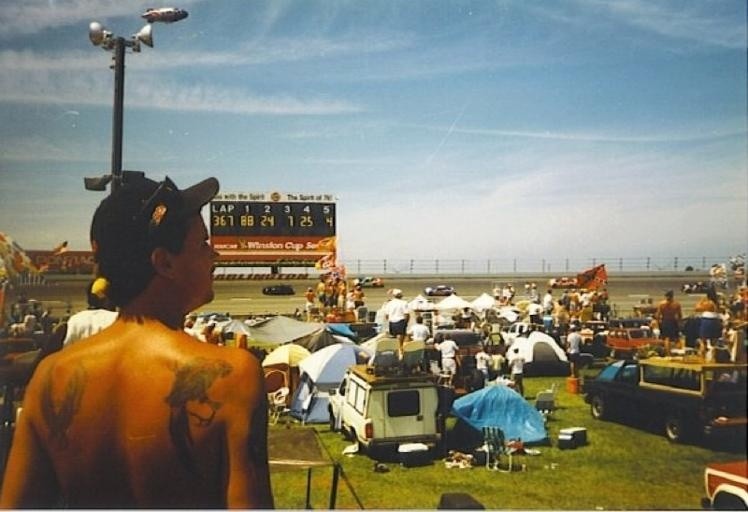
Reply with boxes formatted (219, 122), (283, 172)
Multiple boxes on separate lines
(137, 175), (177, 219)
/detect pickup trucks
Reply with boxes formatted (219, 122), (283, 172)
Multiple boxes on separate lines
(581, 356), (747, 453)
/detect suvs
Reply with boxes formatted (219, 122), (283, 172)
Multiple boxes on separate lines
(496, 292), (709, 359)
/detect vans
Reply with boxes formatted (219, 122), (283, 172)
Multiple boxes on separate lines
(327, 364), (447, 463)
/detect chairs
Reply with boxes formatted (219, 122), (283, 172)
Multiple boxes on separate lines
(266, 386), (291, 425)
(482, 426), (525, 473)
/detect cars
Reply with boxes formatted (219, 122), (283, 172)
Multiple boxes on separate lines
(425, 284), (457, 298)
(699, 460), (747, 511)
(352, 277), (385, 288)
(681, 281), (708, 292)
(548, 277), (580, 288)
(2, 336), (40, 380)
(263, 284), (294, 295)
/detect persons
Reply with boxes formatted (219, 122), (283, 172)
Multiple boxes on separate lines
(0, 273), (608, 461)
(640, 254), (748, 364)
(2, 179), (274, 508)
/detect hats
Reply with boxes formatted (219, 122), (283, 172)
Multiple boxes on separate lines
(393, 289), (403, 297)
(89, 171), (219, 262)
(91, 278), (109, 300)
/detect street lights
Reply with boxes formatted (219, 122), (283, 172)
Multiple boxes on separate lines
(84, 22), (157, 201)
